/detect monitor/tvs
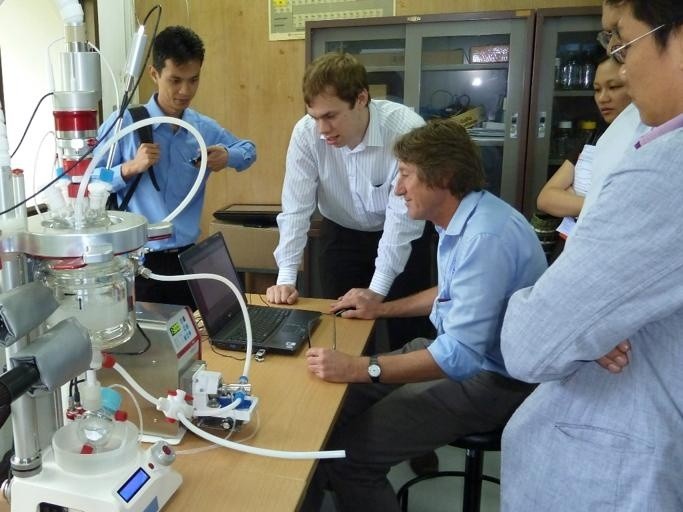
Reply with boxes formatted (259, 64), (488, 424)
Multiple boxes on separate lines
(213, 203), (283, 228)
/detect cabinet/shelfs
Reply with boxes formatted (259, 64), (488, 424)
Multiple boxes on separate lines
(522, 6), (603, 221)
(304, 10), (534, 211)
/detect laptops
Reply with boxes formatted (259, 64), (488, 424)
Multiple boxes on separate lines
(177, 231), (322, 356)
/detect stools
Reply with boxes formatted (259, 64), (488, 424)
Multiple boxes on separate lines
(396, 435), (501, 511)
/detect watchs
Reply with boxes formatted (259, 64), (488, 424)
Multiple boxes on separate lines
(366, 354), (381, 384)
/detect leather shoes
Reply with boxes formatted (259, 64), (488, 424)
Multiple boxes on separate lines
(410, 451), (439, 480)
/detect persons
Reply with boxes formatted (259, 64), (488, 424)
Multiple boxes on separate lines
(533, 45), (635, 263)
(87, 24), (257, 312)
(303, 119), (551, 511)
(498, 0), (683, 511)
(262, 49), (433, 360)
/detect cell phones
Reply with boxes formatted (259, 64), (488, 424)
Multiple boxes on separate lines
(188, 151), (209, 166)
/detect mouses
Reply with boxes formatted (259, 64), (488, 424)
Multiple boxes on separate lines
(334, 306), (355, 317)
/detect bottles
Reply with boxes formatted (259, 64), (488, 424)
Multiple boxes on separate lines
(553, 42), (598, 160)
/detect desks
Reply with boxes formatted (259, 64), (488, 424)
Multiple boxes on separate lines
(144, 293), (377, 511)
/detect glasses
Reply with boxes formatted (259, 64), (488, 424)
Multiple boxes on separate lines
(597, 24), (668, 65)
